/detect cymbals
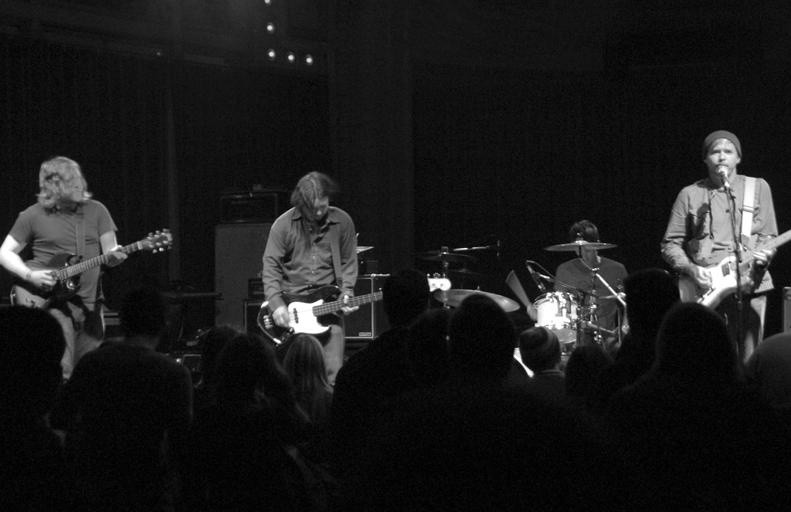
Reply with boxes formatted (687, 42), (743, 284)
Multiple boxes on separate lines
(431, 286), (521, 315)
(545, 236), (619, 254)
(418, 247), (477, 268)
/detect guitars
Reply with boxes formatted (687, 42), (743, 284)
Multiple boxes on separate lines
(256, 284), (394, 347)
(0, 226), (175, 312)
(677, 229), (791, 311)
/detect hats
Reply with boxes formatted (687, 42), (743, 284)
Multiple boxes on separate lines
(701, 130), (742, 160)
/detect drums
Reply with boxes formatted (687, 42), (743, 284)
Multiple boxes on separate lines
(533, 290), (581, 344)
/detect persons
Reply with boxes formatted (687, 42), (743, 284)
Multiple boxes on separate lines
(0, 156), (126, 380)
(1, 267), (331, 510)
(659, 130), (778, 364)
(553, 219), (629, 349)
(262, 172), (359, 394)
(332, 267), (788, 511)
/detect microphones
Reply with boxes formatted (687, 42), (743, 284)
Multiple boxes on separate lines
(714, 165), (734, 189)
